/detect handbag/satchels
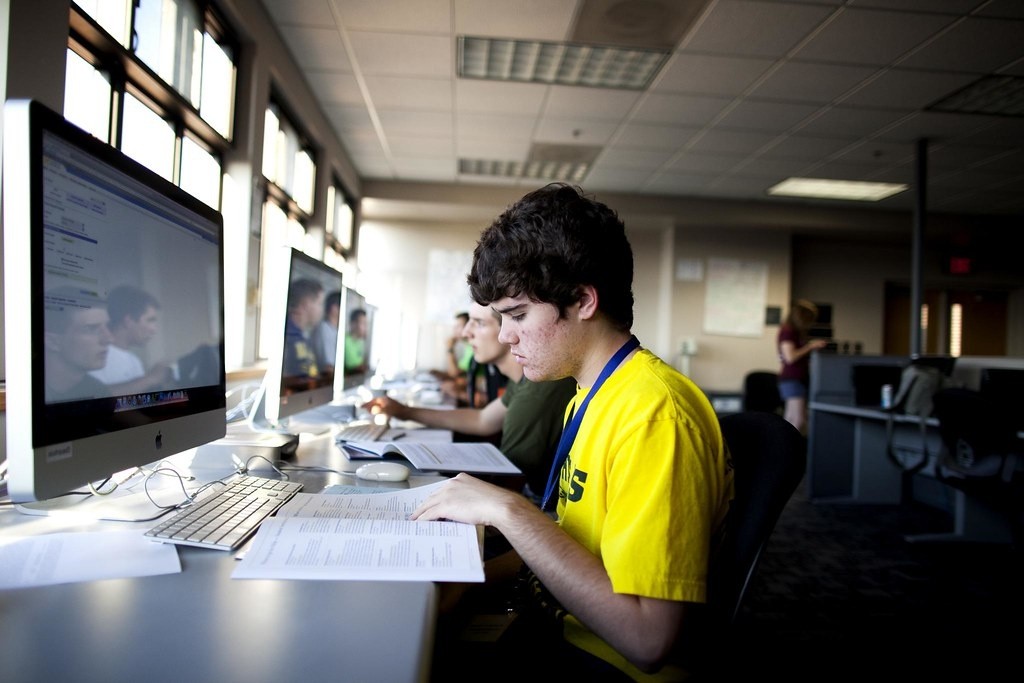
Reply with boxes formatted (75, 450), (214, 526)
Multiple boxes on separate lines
(885, 365), (943, 476)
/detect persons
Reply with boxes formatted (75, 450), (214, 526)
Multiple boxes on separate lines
(363, 301), (576, 524)
(405, 181), (735, 683)
(45, 277), (366, 401)
(777, 305), (826, 429)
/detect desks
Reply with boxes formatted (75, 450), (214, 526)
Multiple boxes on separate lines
(0, 378), (458, 683)
(811, 400), (969, 545)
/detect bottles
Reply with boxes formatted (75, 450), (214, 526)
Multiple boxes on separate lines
(882, 385), (892, 409)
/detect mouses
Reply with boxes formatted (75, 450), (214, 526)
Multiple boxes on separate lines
(421, 391), (443, 403)
(356, 462), (410, 481)
(349, 419), (371, 427)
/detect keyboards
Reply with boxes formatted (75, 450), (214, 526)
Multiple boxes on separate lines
(142, 475), (304, 551)
(335, 424), (387, 444)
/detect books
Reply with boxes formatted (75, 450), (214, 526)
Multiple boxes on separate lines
(232, 438), (523, 583)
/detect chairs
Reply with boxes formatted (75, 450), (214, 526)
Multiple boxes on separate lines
(686, 412), (809, 683)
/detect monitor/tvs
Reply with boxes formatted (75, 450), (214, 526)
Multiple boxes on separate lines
(0, 97), (230, 516)
(247, 245), (379, 436)
